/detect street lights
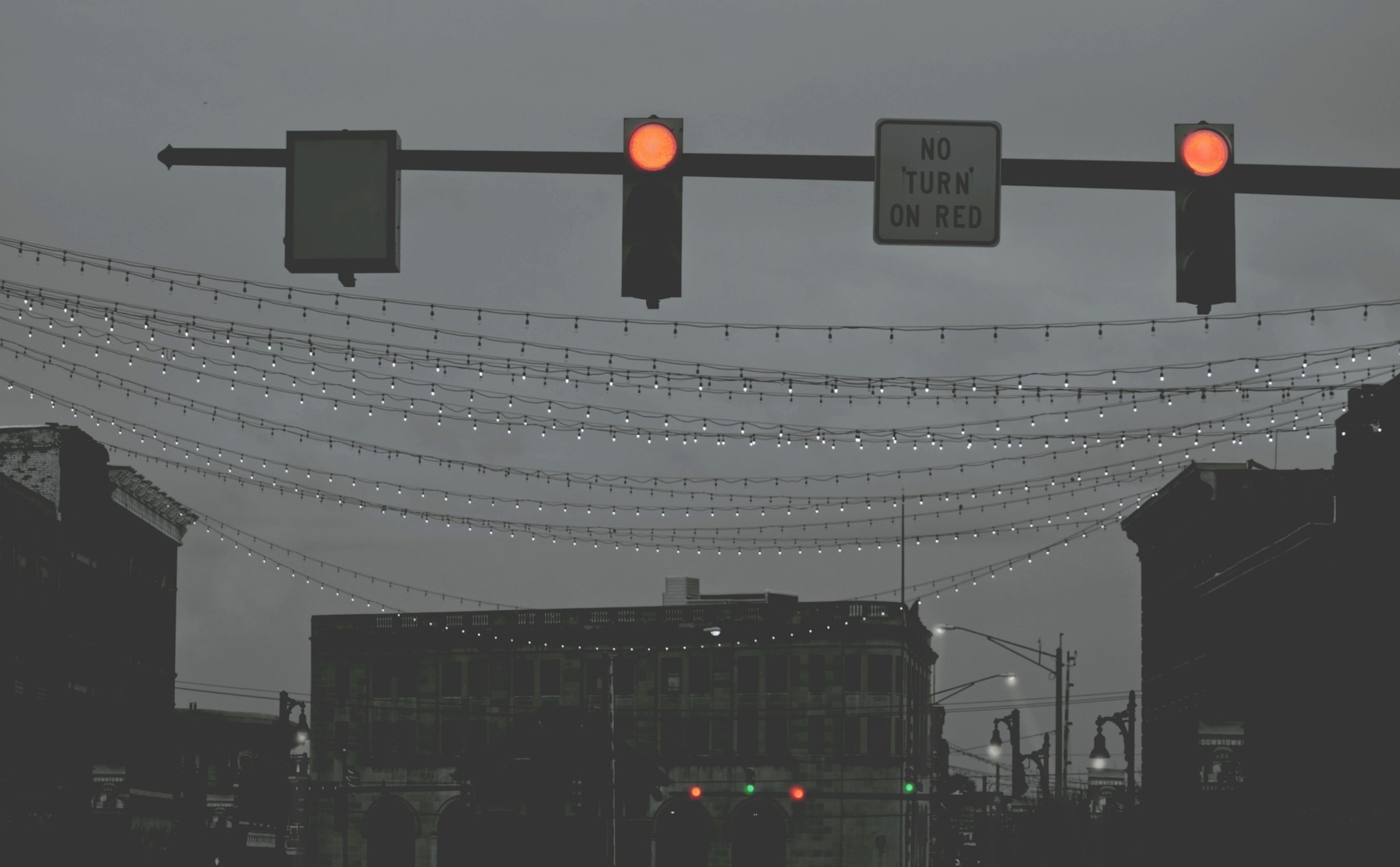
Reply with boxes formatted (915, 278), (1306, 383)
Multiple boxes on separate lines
(934, 624), (1064, 808)
(606, 626), (723, 866)
(1089, 689), (1138, 808)
(275, 690), (311, 867)
(989, 709), (1048, 806)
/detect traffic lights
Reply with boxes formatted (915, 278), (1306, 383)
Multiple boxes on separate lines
(743, 767), (758, 795)
(618, 117), (682, 297)
(902, 764), (917, 795)
(788, 785), (806, 802)
(691, 785), (701, 798)
(1173, 122), (1238, 304)
